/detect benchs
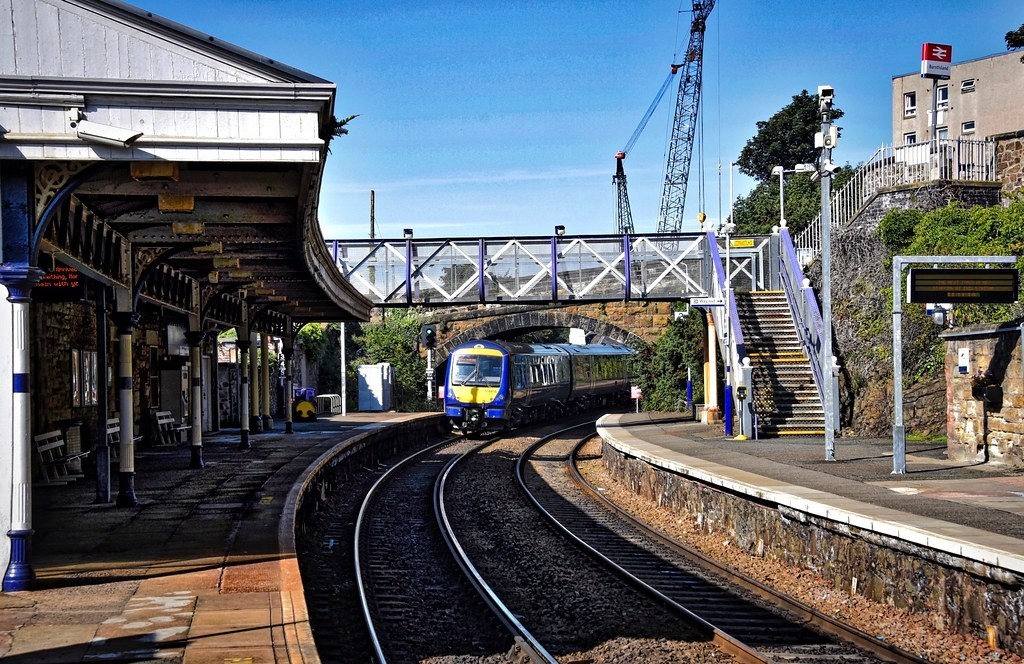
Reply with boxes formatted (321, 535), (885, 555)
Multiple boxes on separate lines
(33, 429), (91, 486)
(105, 417), (143, 458)
(154, 410), (192, 445)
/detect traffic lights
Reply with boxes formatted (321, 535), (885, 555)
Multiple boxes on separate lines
(420, 325), (437, 348)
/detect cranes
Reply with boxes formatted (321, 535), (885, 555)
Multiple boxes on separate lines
(612, 0), (719, 254)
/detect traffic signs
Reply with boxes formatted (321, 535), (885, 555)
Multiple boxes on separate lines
(688, 296), (726, 307)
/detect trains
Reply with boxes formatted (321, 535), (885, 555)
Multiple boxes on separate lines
(445, 339), (645, 440)
